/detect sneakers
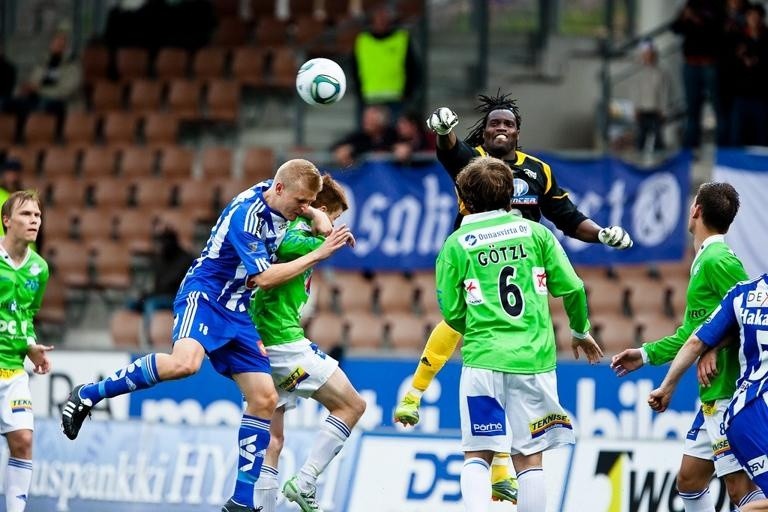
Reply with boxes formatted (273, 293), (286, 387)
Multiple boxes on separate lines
(281, 475), (324, 512)
(393, 391), (421, 427)
(491, 475), (519, 507)
(61, 384), (94, 440)
(221, 497), (263, 512)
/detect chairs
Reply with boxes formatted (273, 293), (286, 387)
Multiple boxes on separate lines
(1, 0), (693, 355)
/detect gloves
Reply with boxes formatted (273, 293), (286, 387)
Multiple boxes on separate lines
(425, 106), (459, 136)
(597, 226), (634, 250)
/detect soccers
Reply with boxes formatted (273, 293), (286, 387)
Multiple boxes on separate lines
(296, 58), (346, 106)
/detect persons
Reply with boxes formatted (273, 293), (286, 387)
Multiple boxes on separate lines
(331, 6), (428, 165)
(611, 1), (767, 151)
(0, 192), (55, 512)
(611, 181), (765, 512)
(105, 4), (220, 79)
(1, 150), (36, 251)
(59, 158), (350, 512)
(394, 85), (633, 502)
(245, 176), (367, 512)
(127, 229), (197, 312)
(434, 154), (603, 512)
(646, 272), (768, 512)
(1, 161), (44, 255)
(14, 33), (83, 143)
